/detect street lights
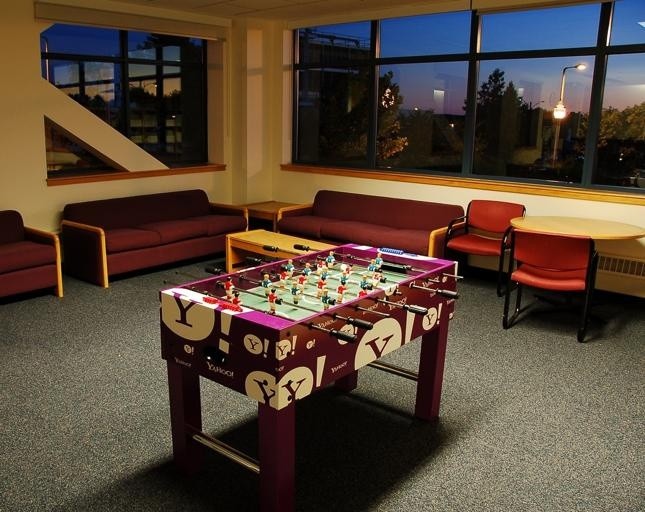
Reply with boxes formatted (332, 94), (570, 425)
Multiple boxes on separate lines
(129, 81), (158, 146)
(528, 100), (545, 149)
(171, 114), (177, 161)
(551, 63), (587, 169)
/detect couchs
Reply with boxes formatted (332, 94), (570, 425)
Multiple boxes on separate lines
(61, 190), (250, 289)
(278, 190), (464, 258)
(0, 209), (63, 298)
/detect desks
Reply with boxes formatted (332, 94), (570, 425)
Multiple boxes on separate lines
(226, 229), (337, 275)
(509, 214), (644, 304)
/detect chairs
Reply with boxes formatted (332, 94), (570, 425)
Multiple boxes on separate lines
(447, 200), (526, 295)
(502, 230), (598, 340)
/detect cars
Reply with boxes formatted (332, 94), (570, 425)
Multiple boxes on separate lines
(618, 169), (645, 188)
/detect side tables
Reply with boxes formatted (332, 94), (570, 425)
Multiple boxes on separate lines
(241, 200), (311, 230)
